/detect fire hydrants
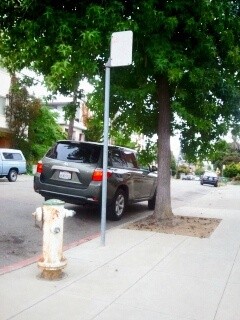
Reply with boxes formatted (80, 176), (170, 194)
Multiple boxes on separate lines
(31, 197), (75, 280)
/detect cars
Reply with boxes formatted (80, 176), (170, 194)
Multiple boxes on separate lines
(201, 172), (219, 186)
(182, 174), (200, 181)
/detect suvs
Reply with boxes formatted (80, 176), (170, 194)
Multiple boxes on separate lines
(0, 148), (27, 182)
(33, 139), (158, 219)
